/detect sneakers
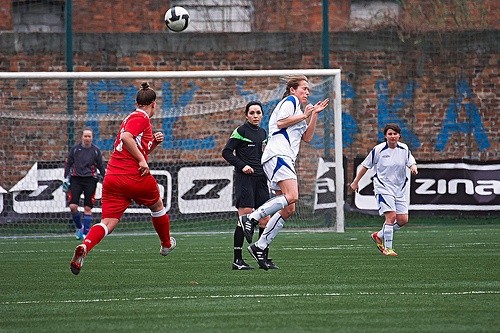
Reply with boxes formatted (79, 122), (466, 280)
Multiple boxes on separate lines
(232, 259), (254, 270)
(75, 225), (87, 240)
(257, 257), (279, 269)
(160, 237), (177, 256)
(248, 241), (280, 271)
(371, 231), (398, 256)
(70, 244), (87, 276)
(239, 213), (259, 244)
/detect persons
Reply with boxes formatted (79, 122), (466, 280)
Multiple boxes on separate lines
(70, 81), (176, 275)
(238, 75), (329, 271)
(221, 100), (280, 270)
(350, 123), (418, 256)
(64, 128), (105, 240)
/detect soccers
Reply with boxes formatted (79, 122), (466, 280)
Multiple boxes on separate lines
(164, 6), (191, 32)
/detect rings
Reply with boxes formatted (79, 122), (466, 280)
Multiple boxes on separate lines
(311, 109), (313, 111)
